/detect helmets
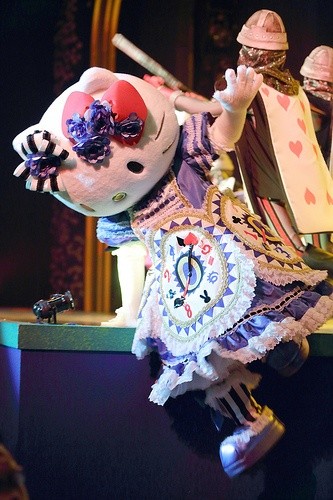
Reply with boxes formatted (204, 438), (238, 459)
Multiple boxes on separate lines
(300, 45), (333, 82)
(237, 9), (289, 50)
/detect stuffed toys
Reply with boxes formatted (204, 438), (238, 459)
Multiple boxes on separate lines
(10, 66), (333, 477)
(215, 7), (333, 255)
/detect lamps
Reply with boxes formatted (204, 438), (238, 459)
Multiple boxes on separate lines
(32, 291), (75, 324)
